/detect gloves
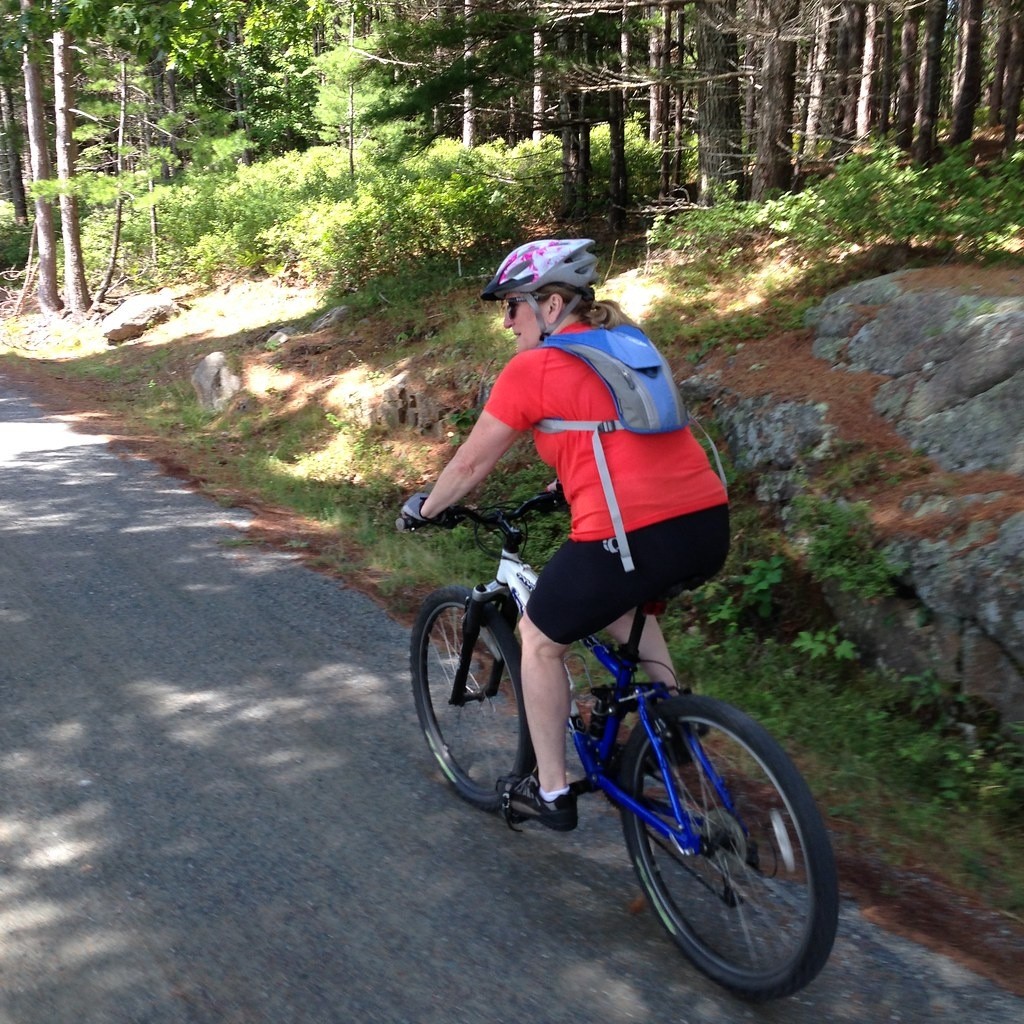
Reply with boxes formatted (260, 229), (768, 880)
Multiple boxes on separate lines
(544, 476), (571, 514)
(399, 491), (441, 531)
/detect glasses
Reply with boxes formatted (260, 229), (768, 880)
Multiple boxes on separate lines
(506, 295), (555, 320)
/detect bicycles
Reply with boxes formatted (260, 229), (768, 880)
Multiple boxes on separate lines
(395, 488), (840, 1002)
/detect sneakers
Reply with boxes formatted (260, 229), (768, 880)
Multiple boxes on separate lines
(644, 733), (693, 768)
(494, 768), (579, 832)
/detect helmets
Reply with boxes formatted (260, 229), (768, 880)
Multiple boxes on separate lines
(479, 238), (598, 302)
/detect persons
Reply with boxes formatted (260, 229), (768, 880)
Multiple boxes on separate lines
(400, 237), (732, 833)
